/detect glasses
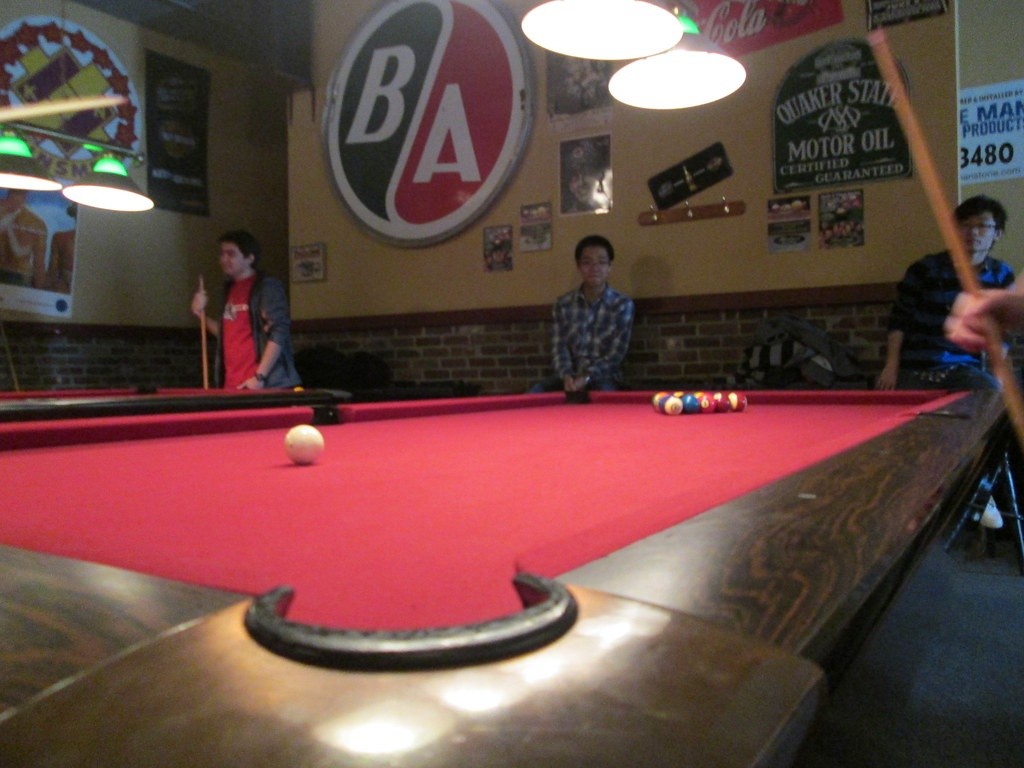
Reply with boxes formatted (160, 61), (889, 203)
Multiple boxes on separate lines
(959, 221), (996, 230)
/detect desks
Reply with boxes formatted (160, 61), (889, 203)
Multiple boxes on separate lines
(0, 379), (1000, 768)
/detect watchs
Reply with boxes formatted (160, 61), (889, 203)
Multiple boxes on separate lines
(255, 372), (266, 381)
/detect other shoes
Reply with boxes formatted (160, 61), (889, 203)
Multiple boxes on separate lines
(980, 496), (1003, 528)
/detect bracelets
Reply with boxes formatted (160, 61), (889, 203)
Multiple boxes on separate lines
(584, 374), (593, 383)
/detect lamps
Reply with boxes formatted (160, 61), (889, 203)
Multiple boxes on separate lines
(0, 122), (155, 211)
(520, 1), (747, 112)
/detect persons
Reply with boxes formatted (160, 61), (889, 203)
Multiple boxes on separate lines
(486, 238), (512, 270)
(192, 230), (301, 389)
(554, 60), (607, 113)
(525, 224), (547, 245)
(877, 195), (1016, 528)
(566, 138), (606, 212)
(551, 234), (633, 394)
(0, 188), (78, 293)
(945, 276), (1024, 352)
